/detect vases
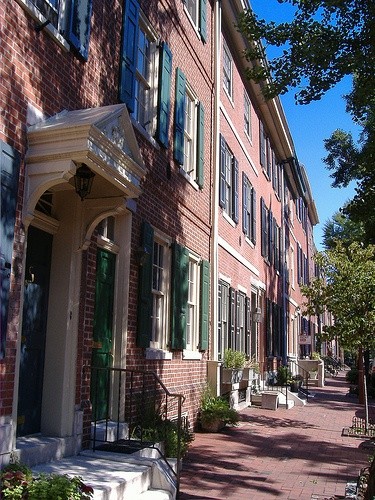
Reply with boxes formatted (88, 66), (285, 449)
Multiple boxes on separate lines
(221, 349), (260, 385)
(196, 398), (238, 431)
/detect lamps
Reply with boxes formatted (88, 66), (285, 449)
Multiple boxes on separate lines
(72, 163), (96, 203)
